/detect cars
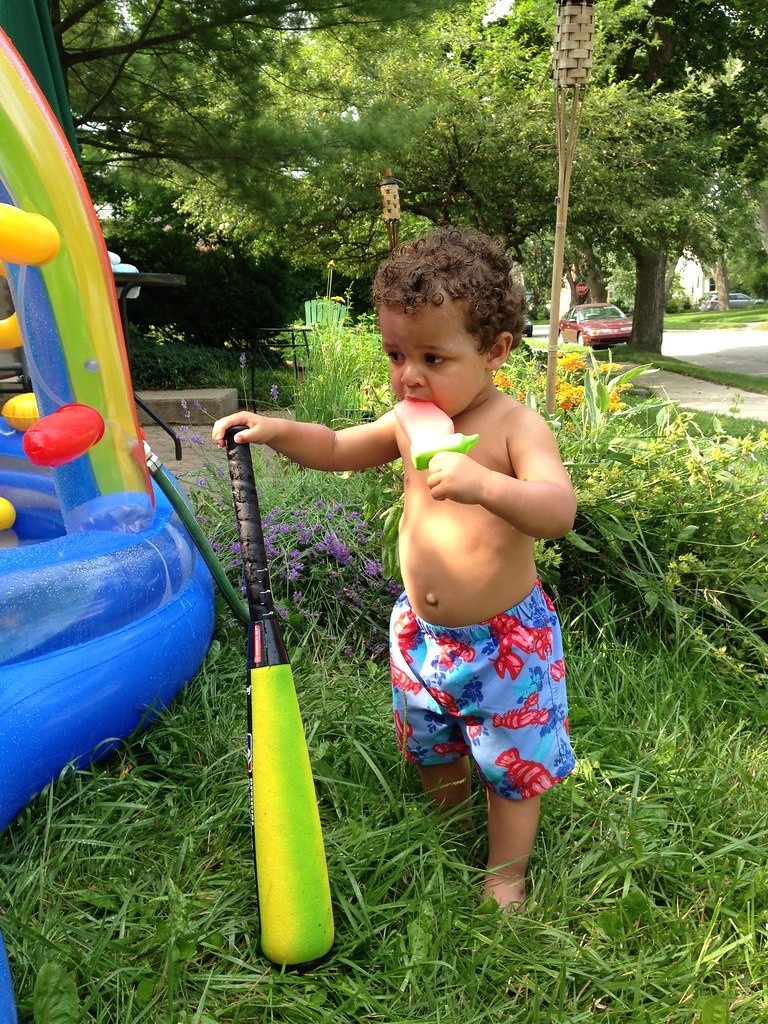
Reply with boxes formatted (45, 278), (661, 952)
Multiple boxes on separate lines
(521, 315), (533, 338)
(699, 291), (765, 313)
(558, 302), (635, 348)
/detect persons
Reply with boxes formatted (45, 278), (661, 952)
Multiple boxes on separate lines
(213, 225), (578, 911)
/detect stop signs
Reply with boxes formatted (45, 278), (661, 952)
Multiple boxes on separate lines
(575, 282), (588, 296)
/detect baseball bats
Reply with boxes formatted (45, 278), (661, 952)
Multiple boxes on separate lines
(216, 425), (340, 967)
